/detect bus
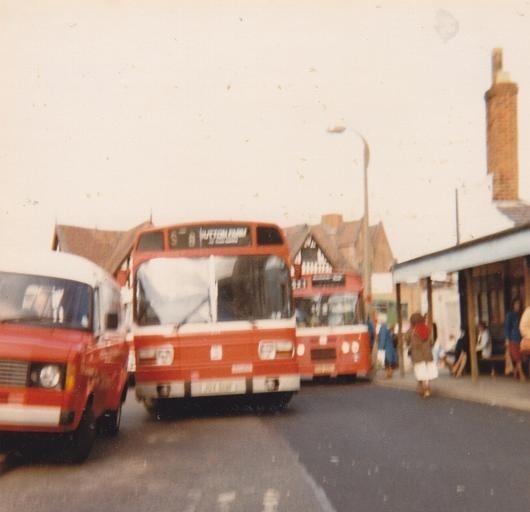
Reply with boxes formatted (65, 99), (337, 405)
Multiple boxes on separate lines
(290, 273), (378, 385)
(0, 245), (131, 467)
(116, 219), (300, 418)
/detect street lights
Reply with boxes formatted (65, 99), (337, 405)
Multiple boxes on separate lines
(329, 122), (375, 384)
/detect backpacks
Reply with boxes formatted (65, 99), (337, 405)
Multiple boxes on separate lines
(414, 323), (430, 343)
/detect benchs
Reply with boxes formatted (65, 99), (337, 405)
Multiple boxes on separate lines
(477, 355), (505, 377)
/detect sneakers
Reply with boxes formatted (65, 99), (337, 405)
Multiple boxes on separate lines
(418, 388), (431, 400)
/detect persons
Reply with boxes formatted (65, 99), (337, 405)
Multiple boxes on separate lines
(366, 312), (377, 372)
(519, 303), (529, 385)
(424, 312), (438, 346)
(379, 313), (397, 380)
(449, 320), (493, 378)
(502, 294), (529, 384)
(296, 300), (312, 327)
(405, 312), (438, 399)
